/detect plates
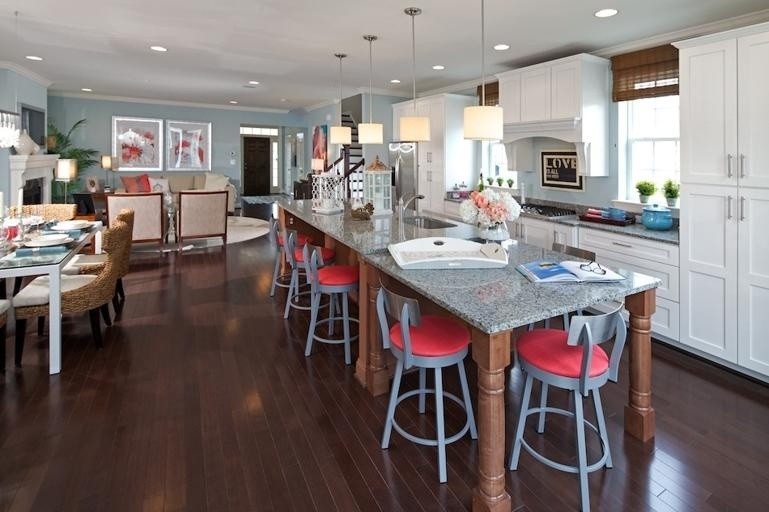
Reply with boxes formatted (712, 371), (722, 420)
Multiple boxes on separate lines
(8, 213), (95, 249)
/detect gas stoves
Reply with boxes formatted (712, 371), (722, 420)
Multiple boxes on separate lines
(518, 203), (577, 219)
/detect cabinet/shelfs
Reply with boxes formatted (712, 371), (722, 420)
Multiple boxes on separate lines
(294, 180), (312, 199)
(390, 92), (480, 213)
(679, 183), (769, 384)
(670, 22), (769, 190)
(578, 228), (680, 345)
(494, 52), (612, 178)
(505, 217), (578, 254)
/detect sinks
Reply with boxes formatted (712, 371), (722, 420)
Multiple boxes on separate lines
(396, 215), (457, 229)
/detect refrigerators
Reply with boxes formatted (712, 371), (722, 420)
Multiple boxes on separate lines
(388, 141), (419, 212)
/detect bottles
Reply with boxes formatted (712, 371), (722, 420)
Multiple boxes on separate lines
(520, 182), (528, 204)
(104, 185), (111, 195)
(459, 181), (468, 198)
(452, 183), (461, 198)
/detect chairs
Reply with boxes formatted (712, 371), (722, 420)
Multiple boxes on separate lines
(376, 275), (478, 483)
(105, 191), (228, 260)
(508, 299), (627, 511)
(1, 203), (135, 374)
(272, 220), (360, 364)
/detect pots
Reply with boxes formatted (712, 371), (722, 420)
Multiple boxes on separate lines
(642, 204), (674, 230)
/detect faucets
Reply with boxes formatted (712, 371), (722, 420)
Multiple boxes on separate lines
(399, 191), (425, 242)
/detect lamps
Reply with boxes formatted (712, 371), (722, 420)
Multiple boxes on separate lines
(310, 159), (324, 175)
(330, 0), (503, 145)
(0, 10), (21, 148)
(55, 158), (78, 203)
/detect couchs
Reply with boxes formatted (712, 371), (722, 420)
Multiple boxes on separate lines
(118, 175), (237, 216)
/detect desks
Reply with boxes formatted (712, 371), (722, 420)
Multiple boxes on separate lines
(273, 199), (488, 386)
(364, 242), (664, 511)
(0, 221), (102, 374)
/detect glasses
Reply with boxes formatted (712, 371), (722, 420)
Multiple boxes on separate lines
(580, 262), (606, 275)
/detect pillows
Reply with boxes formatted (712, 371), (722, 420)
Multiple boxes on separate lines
(120, 173), (230, 193)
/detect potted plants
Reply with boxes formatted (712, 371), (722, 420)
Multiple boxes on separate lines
(47, 119), (100, 204)
(486, 176), (514, 188)
(661, 179), (680, 206)
(636, 181), (658, 203)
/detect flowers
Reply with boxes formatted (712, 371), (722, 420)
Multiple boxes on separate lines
(460, 187), (521, 227)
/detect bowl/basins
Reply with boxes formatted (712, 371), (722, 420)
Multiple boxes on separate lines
(600, 207), (626, 221)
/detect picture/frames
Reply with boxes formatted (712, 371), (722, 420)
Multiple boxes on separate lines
(539, 152), (585, 193)
(111, 116), (163, 171)
(166, 118), (212, 172)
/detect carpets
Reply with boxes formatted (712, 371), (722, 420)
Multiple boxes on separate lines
(244, 195), (289, 205)
(131, 216), (270, 253)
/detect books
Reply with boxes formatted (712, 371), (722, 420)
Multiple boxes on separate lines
(515, 260), (628, 283)
